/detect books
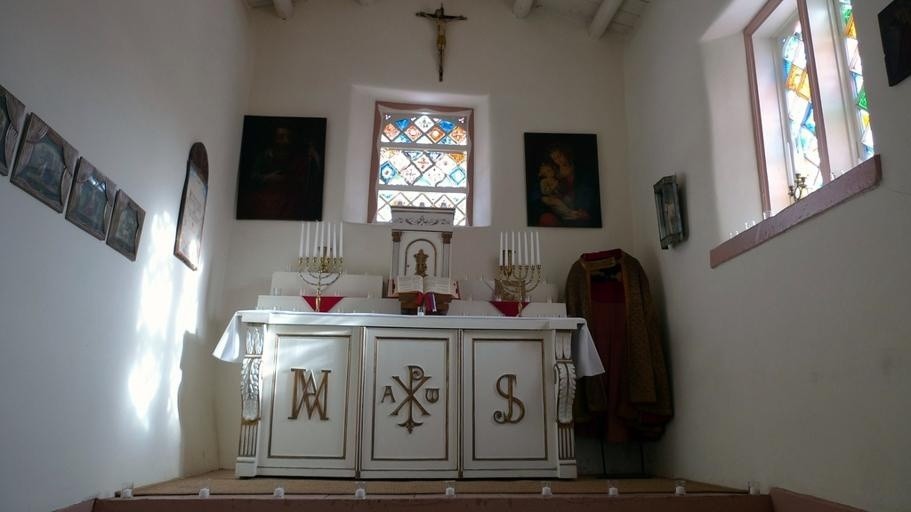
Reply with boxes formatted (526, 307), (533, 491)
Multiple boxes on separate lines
(536, 138), (591, 223)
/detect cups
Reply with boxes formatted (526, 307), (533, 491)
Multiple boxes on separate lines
(674, 479), (688, 496)
(746, 221), (755, 229)
(540, 481), (552, 496)
(729, 230), (738, 239)
(121, 480), (134, 498)
(353, 481), (366, 499)
(198, 478), (212, 499)
(417, 305), (427, 316)
(763, 210), (774, 220)
(271, 481), (285, 498)
(444, 479), (456, 498)
(748, 480), (760, 496)
(607, 480), (619, 497)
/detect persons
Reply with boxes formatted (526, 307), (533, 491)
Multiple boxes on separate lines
(241, 121), (324, 220)
(396, 274), (457, 298)
(536, 161), (588, 222)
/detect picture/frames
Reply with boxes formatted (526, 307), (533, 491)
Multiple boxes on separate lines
(0, 84), (146, 262)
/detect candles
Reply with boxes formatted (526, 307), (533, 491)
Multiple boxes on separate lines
(499, 231), (540, 266)
(123, 488), (133, 497)
(610, 488), (618, 496)
(785, 132), (806, 186)
(299, 220), (343, 258)
(355, 489), (365, 496)
(446, 488), (456, 496)
(542, 488), (551, 495)
(676, 488), (684, 495)
(750, 486), (760, 497)
(199, 489), (211, 496)
(274, 489), (285, 496)
